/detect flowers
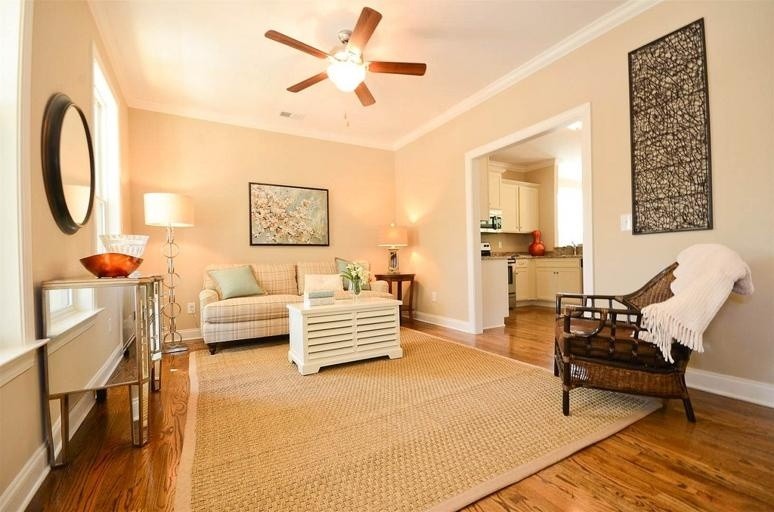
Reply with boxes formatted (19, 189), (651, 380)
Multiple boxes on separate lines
(339, 262), (367, 290)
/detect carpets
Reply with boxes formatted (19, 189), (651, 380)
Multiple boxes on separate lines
(172, 321), (665, 512)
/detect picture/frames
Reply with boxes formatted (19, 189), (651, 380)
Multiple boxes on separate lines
(248, 180), (331, 248)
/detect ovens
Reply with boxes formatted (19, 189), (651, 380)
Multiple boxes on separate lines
(508, 258), (516, 310)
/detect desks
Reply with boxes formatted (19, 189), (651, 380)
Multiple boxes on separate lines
(373, 273), (417, 327)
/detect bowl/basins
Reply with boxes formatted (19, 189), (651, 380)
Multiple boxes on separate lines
(78, 232), (150, 279)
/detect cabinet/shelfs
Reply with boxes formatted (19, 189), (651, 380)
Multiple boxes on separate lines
(40, 275), (166, 469)
(480, 156), (489, 222)
(501, 179), (540, 234)
(489, 163), (506, 210)
(516, 258), (533, 308)
(534, 257), (583, 308)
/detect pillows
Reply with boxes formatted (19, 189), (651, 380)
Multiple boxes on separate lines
(206, 265), (268, 302)
(334, 256), (371, 292)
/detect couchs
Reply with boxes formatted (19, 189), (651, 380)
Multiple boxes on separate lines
(190, 259), (389, 356)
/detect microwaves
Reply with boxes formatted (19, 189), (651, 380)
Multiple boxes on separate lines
(480, 209), (503, 233)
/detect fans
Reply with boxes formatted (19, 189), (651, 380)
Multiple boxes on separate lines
(260, 7), (427, 106)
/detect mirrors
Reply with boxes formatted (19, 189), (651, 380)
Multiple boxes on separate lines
(40, 92), (96, 237)
(46, 281), (163, 467)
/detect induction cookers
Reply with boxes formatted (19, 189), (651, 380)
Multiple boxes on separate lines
(490, 251), (515, 257)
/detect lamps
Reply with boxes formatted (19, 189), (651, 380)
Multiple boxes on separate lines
(377, 222), (408, 273)
(325, 52), (365, 95)
(141, 190), (197, 357)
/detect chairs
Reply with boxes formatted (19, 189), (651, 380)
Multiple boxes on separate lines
(553, 244), (754, 424)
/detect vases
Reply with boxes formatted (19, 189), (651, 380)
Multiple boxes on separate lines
(347, 280), (360, 295)
